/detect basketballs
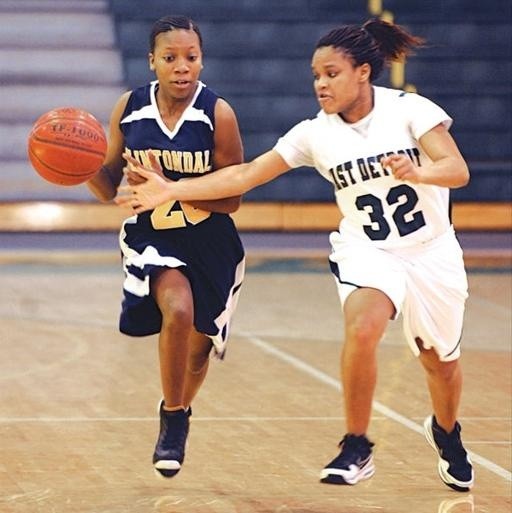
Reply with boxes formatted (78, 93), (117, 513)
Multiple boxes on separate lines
(28, 108), (107, 186)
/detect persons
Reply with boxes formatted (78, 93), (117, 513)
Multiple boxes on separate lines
(82, 12), (249, 483)
(109, 12), (475, 494)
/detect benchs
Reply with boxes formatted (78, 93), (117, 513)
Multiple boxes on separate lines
(1, 2), (512, 206)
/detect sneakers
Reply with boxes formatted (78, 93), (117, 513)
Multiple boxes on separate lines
(153, 400), (192, 478)
(424, 417), (474, 492)
(320, 433), (375, 484)
